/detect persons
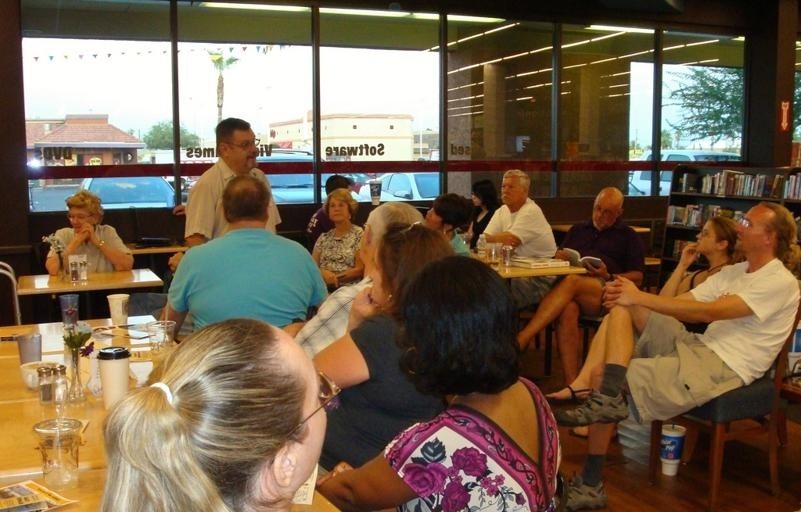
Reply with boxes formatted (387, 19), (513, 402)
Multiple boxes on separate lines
(424, 170), (557, 310)
(102, 316), (329, 511)
(305, 176), (364, 287)
(312, 223), (454, 470)
(157, 174), (327, 342)
(546, 201), (801, 511)
(173, 203), (186, 216)
(282, 201), (423, 361)
(184, 117), (284, 247)
(517, 187), (646, 388)
(44, 189), (134, 321)
(555, 200), (801, 511)
(317, 250), (564, 511)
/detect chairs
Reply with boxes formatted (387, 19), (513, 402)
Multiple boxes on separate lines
(1, 222), (801, 511)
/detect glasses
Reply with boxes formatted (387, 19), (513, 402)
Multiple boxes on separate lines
(738, 217), (751, 228)
(270, 371), (342, 466)
(224, 139), (260, 150)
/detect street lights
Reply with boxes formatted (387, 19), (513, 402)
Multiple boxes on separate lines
(415, 126), (433, 157)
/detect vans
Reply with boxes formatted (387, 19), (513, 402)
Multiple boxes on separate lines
(629, 150), (741, 197)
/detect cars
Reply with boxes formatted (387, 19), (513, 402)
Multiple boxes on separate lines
(79, 176), (177, 209)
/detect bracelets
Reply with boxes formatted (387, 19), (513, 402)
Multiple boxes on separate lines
(95, 239), (106, 249)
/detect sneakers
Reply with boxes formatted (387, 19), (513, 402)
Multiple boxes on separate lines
(553, 388), (629, 427)
(563, 480), (608, 512)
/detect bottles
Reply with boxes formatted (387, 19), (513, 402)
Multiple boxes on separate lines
(476, 233), (488, 262)
(70, 261), (88, 282)
(491, 243), (496, 261)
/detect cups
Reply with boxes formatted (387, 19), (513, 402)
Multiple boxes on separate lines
(105, 293), (130, 325)
(58, 294), (79, 326)
(786, 320), (800, 374)
(500, 245), (513, 267)
(20, 361), (56, 392)
(145, 320), (177, 369)
(660, 423), (687, 476)
(37, 365), (69, 405)
(16, 333), (42, 364)
(369, 180), (382, 205)
(32, 418), (83, 492)
(96, 345), (131, 410)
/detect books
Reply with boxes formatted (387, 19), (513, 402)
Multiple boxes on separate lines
(511, 248), (601, 269)
(661, 165), (801, 376)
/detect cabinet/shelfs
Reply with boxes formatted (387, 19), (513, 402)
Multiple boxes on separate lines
(654, 163), (799, 399)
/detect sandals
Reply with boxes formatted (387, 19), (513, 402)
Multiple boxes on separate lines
(548, 385), (592, 404)
